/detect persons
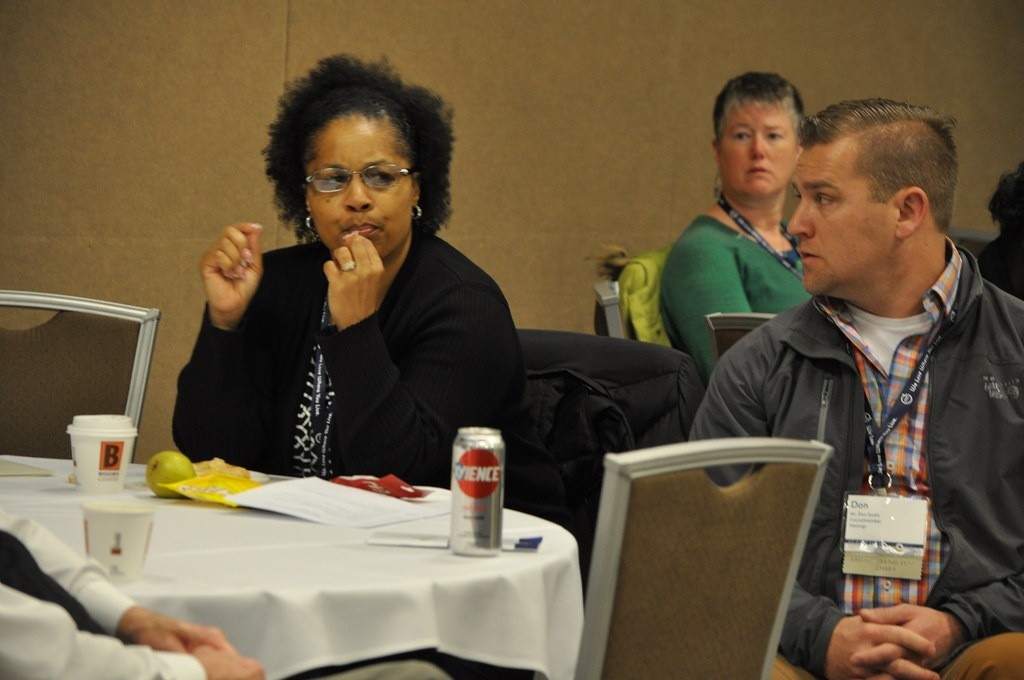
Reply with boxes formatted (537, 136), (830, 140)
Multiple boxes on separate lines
(687, 96), (1022, 680)
(170, 54), (521, 511)
(663, 71), (814, 382)
(1, 518), (265, 678)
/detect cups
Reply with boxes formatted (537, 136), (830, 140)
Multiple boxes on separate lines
(81, 500), (154, 583)
(66, 414), (138, 495)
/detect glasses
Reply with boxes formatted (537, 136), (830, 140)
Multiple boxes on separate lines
(305, 161), (413, 194)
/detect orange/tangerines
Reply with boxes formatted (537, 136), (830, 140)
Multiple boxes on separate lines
(145, 450), (196, 498)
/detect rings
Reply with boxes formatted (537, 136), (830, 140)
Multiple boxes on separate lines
(339, 260), (355, 271)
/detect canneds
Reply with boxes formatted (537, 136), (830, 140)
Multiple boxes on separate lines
(449, 427), (505, 557)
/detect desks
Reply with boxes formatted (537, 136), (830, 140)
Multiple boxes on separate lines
(0, 454), (584, 680)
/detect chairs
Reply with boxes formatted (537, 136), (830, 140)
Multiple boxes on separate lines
(0, 290), (162, 463)
(514, 328), (704, 603)
(575, 438), (832, 679)
